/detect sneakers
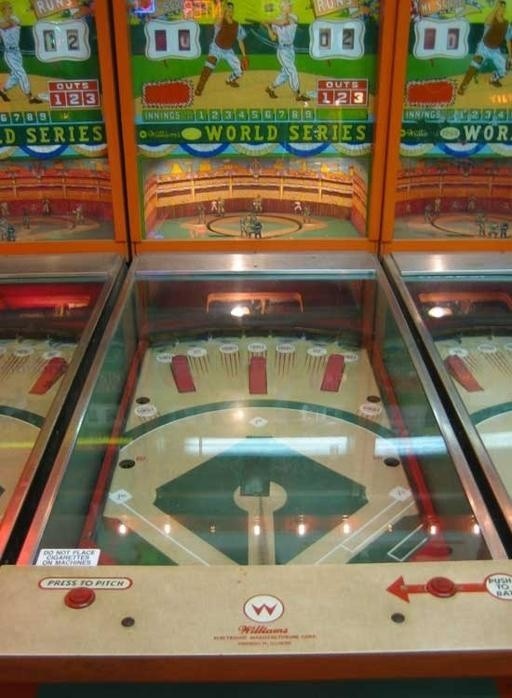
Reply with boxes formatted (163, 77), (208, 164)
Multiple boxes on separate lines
(296, 95), (311, 101)
(195, 86), (204, 96)
(29, 98), (42, 104)
(0, 90), (10, 101)
(265, 88), (277, 98)
(226, 80), (239, 87)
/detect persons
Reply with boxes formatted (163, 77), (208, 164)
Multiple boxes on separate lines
(1, 2), (47, 103)
(198, 3), (311, 100)
